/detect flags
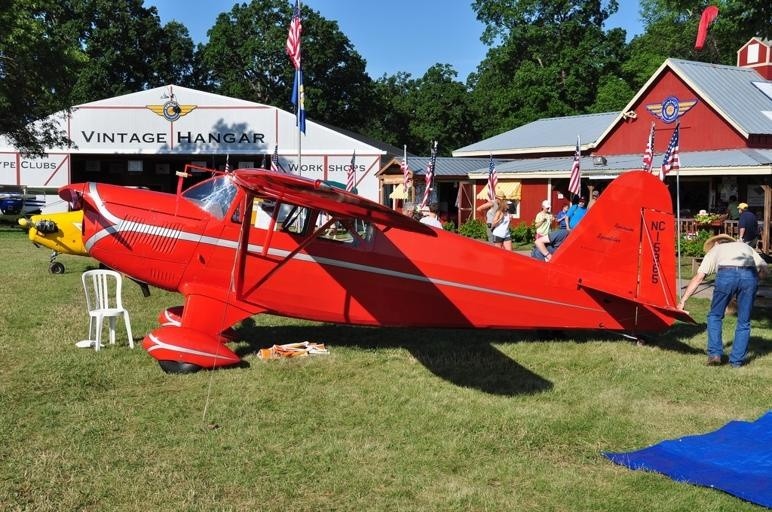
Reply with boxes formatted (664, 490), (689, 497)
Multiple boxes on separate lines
(660, 121), (680, 181)
(286, 0), (301, 67)
(642, 122), (655, 174)
(419, 144), (435, 207)
(487, 155), (499, 201)
(291, 67), (306, 137)
(568, 137), (581, 198)
(401, 149), (410, 193)
(346, 152), (355, 194)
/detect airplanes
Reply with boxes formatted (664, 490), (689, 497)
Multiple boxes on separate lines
(0, 183), (151, 227)
(58, 158), (701, 374)
(19, 196), (271, 275)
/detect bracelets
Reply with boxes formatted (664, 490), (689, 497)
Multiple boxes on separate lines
(542, 252), (551, 257)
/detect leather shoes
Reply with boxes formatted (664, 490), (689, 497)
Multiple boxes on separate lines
(704, 355), (722, 365)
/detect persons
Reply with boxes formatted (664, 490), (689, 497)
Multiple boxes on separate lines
(529, 230), (572, 263)
(736, 202), (758, 248)
(484, 194), (505, 245)
(492, 199), (513, 253)
(565, 198), (587, 232)
(676, 233), (768, 368)
(534, 199), (556, 240)
(587, 189), (599, 209)
(419, 203), (443, 230)
(725, 195), (740, 220)
(556, 203), (568, 230)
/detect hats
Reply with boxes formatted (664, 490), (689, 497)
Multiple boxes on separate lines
(592, 190), (599, 197)
(703, 233), (736, 253)
(736, 202), (749, 211)
(419, 206), (429, 211)
(505, 200), (512, 204)
(495, 191), (506, 198)
(541, 199), (551, 208)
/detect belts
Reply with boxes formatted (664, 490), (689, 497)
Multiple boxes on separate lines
(718, 265), (754, 270)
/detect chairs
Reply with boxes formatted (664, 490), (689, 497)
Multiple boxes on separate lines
(82, 270), (136, 353)
